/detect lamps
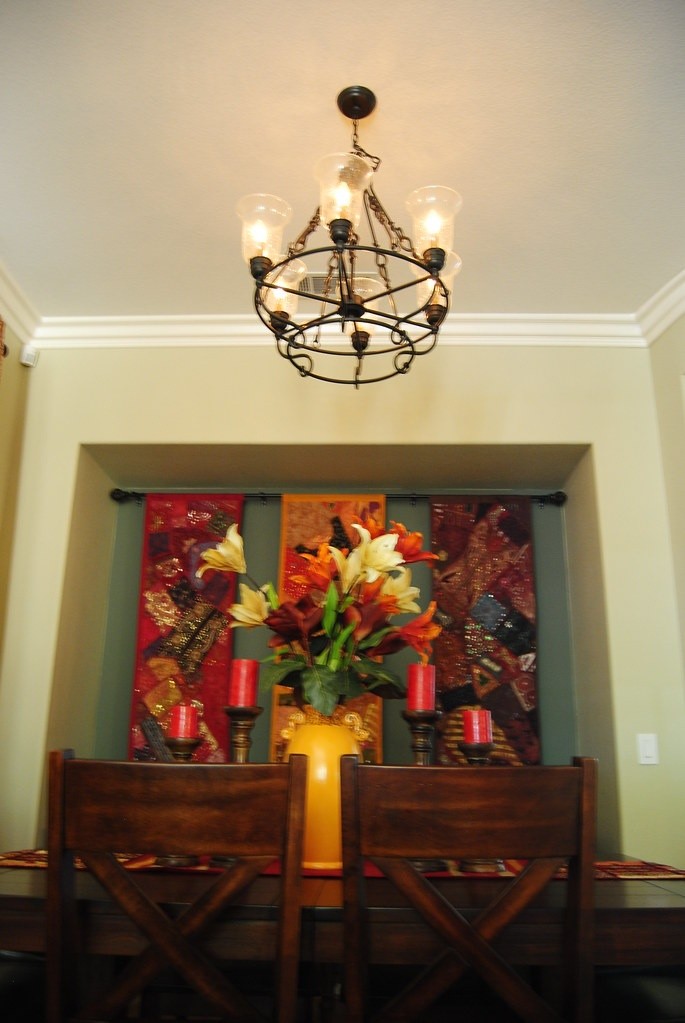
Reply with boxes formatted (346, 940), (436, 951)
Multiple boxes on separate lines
(234, 85), (466, 389)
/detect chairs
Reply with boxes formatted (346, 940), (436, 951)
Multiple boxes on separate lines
(339, 755), (598, 1023)
(42, 745), (309, 1022)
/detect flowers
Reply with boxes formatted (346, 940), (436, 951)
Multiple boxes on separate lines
(196, 513), (442, 718)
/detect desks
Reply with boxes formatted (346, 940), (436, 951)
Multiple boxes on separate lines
(1, 848), (684, 1023)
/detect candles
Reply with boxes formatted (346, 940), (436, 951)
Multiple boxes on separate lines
(167, 705), (200, 741)
(461, 710), (493, 744)
(404, 662), (437, 711)
(225, 659), (259, 709)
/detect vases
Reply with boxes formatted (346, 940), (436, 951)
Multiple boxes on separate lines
(278, 712), (375, 869)
